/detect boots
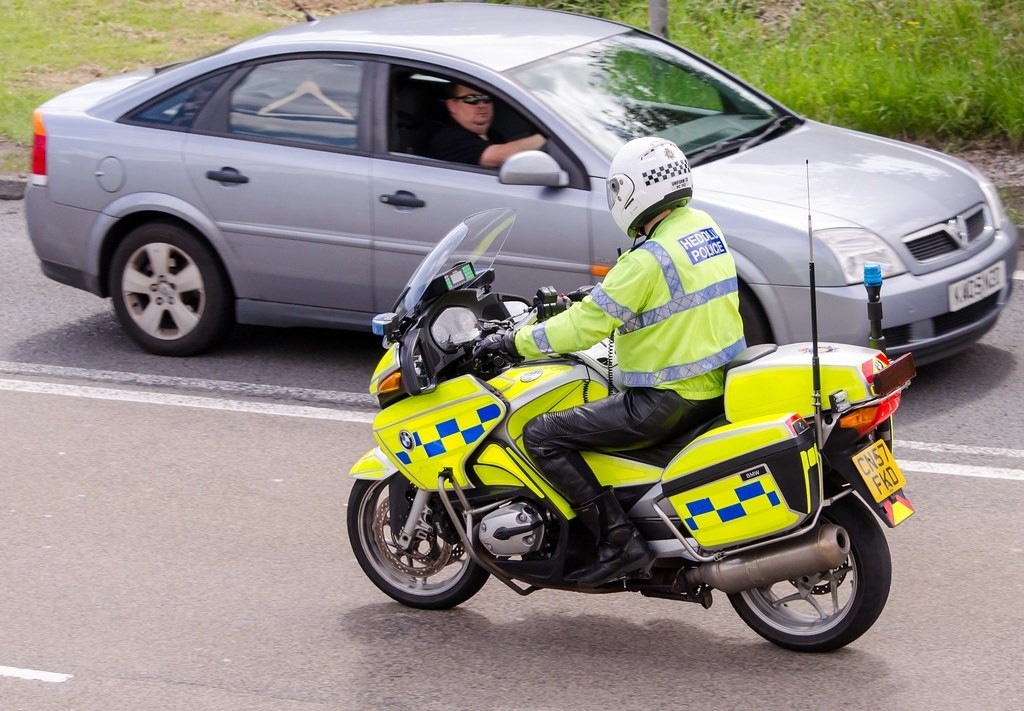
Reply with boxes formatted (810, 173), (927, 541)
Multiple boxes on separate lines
(563, 485), (659, 587)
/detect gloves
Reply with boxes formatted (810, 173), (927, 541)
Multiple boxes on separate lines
(566, 284), (594, 301)
(470, 328), (525, 375)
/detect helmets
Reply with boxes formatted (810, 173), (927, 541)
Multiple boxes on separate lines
(606, 136), (693, 239)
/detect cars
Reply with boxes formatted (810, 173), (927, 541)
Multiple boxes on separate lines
(25, 3), (1019, 365)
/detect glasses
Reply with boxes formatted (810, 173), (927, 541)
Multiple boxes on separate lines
(440, 92), (492, 105)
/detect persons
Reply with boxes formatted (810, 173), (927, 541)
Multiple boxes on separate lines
(423, 77), (547, 169)
(473, 137), (747, 589)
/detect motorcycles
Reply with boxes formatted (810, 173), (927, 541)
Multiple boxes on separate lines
(346, 209), (917, 652)
